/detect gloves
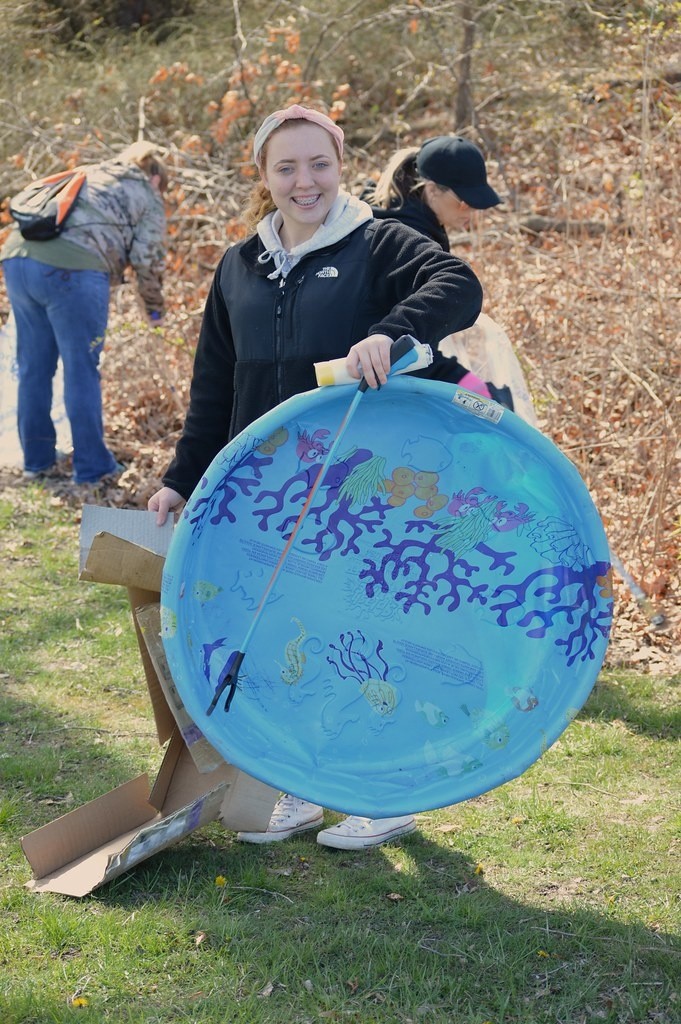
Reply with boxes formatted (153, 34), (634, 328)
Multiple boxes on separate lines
(456, 371), (492, 400)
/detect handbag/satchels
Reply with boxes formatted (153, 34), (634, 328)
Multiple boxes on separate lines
(484, 382), (514, 412)
(439, 312), (538, 432)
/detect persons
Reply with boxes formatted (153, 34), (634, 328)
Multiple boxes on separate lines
(359, 136), (514, 412)
(147, 104), (483, 850)
(0, 140), (169, 486)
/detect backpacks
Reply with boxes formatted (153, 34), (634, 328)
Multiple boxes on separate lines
(8, 168), (137, 240)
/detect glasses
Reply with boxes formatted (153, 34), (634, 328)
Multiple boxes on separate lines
(439, 184), (478, 212)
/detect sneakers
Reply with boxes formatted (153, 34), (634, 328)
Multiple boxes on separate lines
(237, 794), (324, 843)
(317, 814), (416, 850)
(22, 449), (68, 478)
(72, 463), (127, 489)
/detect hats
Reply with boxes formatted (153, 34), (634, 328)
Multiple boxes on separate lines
(417, 136), (500, 209)
